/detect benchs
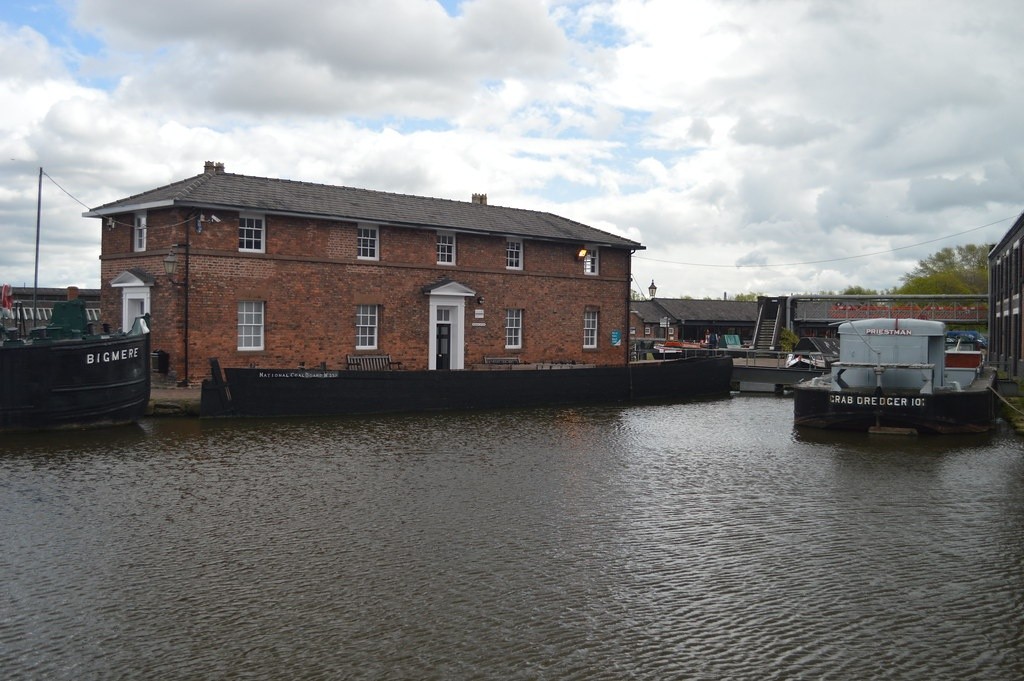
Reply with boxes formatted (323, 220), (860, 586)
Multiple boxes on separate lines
(345, 354), (402, 369)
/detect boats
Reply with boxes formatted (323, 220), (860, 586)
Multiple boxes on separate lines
(198, 350), (734, 422)
(783, 319), (1001, 441)
(0, 285), (150, 434)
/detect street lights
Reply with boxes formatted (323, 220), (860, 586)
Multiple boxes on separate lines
(648, 280), (657, 299)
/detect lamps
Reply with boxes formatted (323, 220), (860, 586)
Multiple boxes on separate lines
(576, 247), (587, 261)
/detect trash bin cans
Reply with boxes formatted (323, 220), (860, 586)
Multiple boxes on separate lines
(150, 349), (169, 374)
